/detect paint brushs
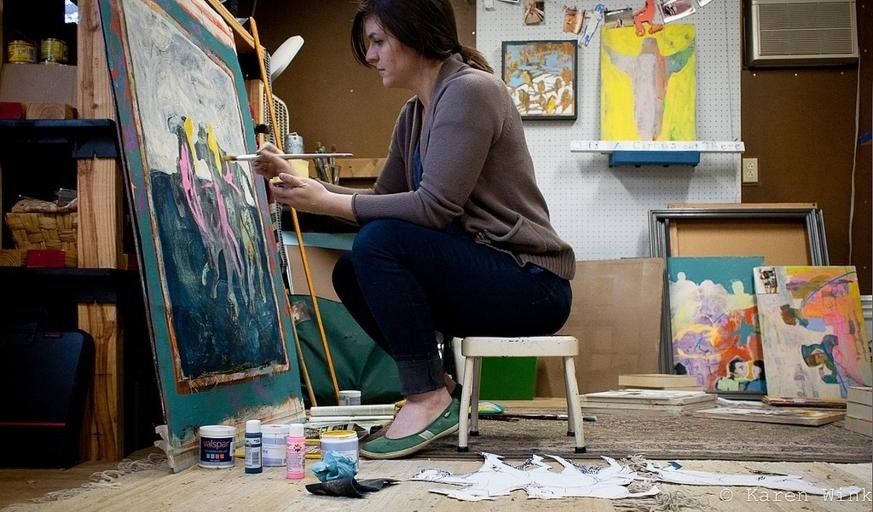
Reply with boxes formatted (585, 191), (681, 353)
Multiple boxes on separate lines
(468, 412), (597, 422)
(224, 153), (354, 160)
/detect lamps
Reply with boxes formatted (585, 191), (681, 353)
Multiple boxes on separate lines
(267, 35), (304, 87)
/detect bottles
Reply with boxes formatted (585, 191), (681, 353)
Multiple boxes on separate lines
(197, 425), (236, 469)
(284, 422), (308, 479)
(243, 419), (262, 474)
(320, 430), (360, 478)
(262, 424), (286, 467)
(337, 390), (361, 406)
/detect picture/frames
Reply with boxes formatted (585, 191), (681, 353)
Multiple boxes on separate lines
(650, 207), (831, 375)
(501, 40), (577, 121)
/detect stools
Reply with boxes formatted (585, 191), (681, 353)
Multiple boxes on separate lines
(458, 334), (584, 451)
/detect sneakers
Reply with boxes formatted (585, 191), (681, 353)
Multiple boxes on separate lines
(358, 382), (470, 462)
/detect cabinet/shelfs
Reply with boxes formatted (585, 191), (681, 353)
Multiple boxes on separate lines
(0, 0), (144, 465)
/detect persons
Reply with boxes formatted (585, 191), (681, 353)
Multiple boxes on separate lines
(253, 0), (577, 460)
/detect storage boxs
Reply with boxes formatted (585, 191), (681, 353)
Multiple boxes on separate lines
(334, 157), (389, 189)
